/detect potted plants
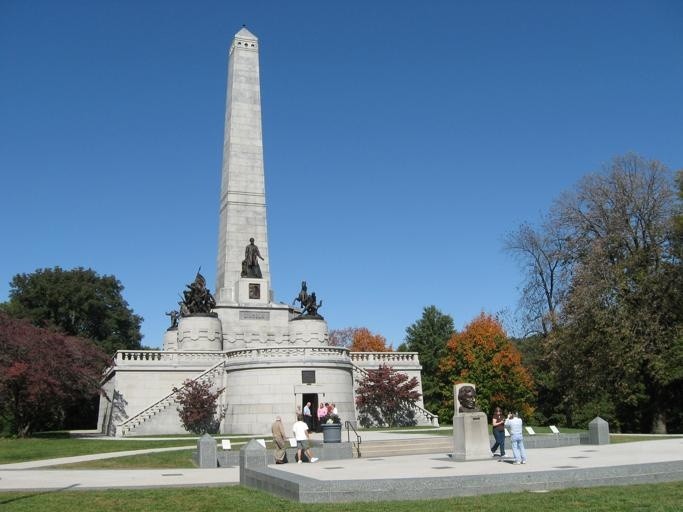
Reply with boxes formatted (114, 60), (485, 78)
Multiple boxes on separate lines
(321, 414), (341, 443)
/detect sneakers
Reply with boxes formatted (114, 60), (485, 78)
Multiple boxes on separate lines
(276, 460), (284, 464)
(311, 458), (319, 462)
(492, 450), (509, 458)
(512, 460), (527, 465)
(298, 460), (302, 464)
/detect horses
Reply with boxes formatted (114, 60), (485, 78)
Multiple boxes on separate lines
(292, 280), (315, 316)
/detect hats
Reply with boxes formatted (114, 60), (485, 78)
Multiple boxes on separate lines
(276, 415), (281, 420)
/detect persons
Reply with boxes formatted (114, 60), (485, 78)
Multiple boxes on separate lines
(295, 404), (302, 416)
(504, 410), (526, 466)
(331, 404), (339, 423)
(164, 271), (216, 330)
(324, 402), (334, 417)
(316, 402), (327, 432)
(303, 402), (312, 434)
(310, 291), (318, 316)
(490, 407), (507, 458)
(243, 237), (263, 275)
(270, 415), (288, 464)
(291, 414), (319, 464)
(457, 385), (475, 409)
